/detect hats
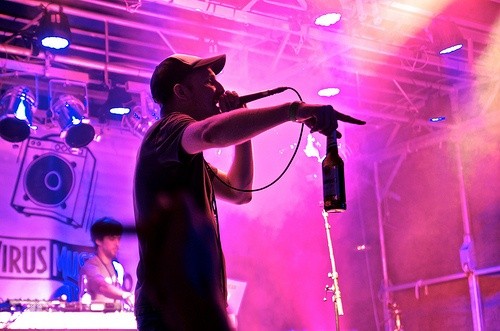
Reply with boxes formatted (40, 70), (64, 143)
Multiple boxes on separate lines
(149, 53), (226, 103)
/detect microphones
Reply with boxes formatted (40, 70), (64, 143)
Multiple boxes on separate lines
(225, 87), (287, 110)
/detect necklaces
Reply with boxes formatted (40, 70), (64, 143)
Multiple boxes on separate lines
(94, 252), (117, 303)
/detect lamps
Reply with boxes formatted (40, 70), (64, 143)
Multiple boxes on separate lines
(430, 8), (465, 56)
(0, 71), (38, 142)
(104, 85), (156, 138)
(288, 0), (342, 32)
(48, 79), (96, 149)
(35, 4), (72, 52)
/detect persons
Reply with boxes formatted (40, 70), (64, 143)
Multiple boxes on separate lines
(79, 218), (135, 307)
(133, 54), (366, 331)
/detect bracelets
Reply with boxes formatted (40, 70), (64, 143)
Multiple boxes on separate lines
(289, 101), (305, 123)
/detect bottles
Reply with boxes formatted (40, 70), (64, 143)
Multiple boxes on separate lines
(80, 275), (92, 311)
(322, 128), (346, 214)
